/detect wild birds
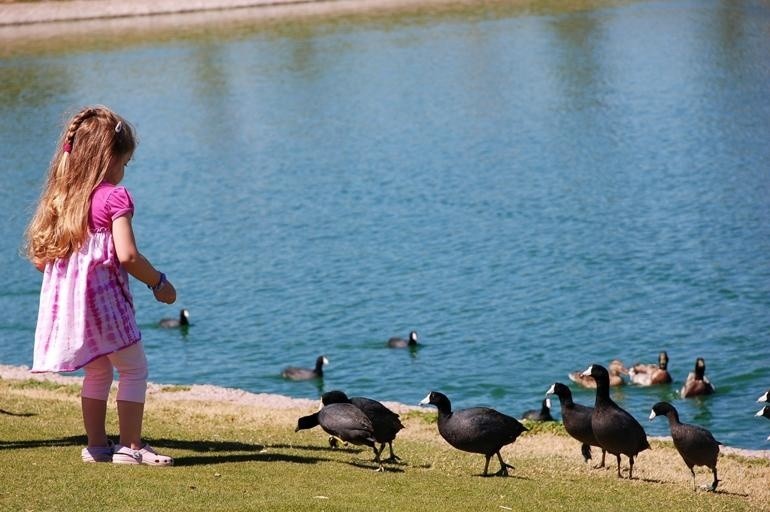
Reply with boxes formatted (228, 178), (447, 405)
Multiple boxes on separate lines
(294, 390), (406, 474)
(523, 398), (556, 421)
(158, 308), (190, 328)
(647, 401), (724, 493)
(417, 390), (530, 477)
(546, 363), (652, 480)
(388, 331), (419, 348)
(281, 354), (330, 381)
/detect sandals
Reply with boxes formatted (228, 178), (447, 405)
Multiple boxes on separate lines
(81, 439), (174, 466)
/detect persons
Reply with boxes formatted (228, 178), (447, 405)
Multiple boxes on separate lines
(19, 102), (177, 469)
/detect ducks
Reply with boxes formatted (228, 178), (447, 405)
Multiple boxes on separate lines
(679, 357), (716, 399)
(566, 359), (630, 390)
(627, 350), (673, 387)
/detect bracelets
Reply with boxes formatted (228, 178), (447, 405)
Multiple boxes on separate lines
(145, 270), (167, 292)
